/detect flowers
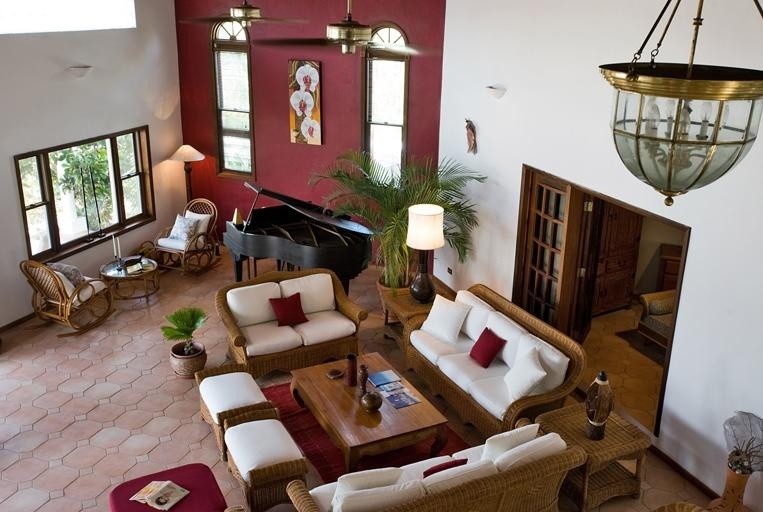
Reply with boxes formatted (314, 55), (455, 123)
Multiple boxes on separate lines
(722, 445), (756, 476)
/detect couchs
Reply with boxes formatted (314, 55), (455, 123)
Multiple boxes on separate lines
(402, 284), (587, 438)
(286, 427), (589, 511)
(212, 268), (368, 380)
(639, 290), (679, 351)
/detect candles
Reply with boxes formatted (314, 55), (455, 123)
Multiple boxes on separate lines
(112, 235), (117, 257)
(117, 237), (121, 258)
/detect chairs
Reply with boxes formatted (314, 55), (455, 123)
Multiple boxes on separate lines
(18, 258), (114, 338)
(150, 196), (218, 278)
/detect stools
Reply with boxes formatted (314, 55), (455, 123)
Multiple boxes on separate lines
(107, 462), (227, 511)
(221, 407), (307, 512)
(195, 363), (280, 463)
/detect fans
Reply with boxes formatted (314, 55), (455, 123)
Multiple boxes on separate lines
(252, 1), (461, 68)
(179, 1), (314, 34)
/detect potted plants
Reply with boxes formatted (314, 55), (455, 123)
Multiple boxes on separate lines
(155, 305), (211, 383)
(304, 148), (488, 320)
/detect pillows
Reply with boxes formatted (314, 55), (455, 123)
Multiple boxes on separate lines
(482, 421), (541, 466)
(268, 293), (309, 328)
(419, 293), (473, 343)
(46, 261), (87, 289)
(184, 210), (213, 242)
(470, 326), (508, 368)
(329, 461), (405, 508)
(422, 457), (469, 479)
(170, 212), (201, 240)
(502, 347), (548, 403)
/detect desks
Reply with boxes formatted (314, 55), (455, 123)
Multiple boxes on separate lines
(377, 283), (459, 347)
(532, 402), (651, 511)
(96, 256), (162, 303)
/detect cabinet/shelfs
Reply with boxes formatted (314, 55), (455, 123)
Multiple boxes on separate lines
(591, 203), (646, 316)
(659, 242), (681, 291)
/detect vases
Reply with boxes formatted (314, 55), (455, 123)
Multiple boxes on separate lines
(702, 463), (753, 512)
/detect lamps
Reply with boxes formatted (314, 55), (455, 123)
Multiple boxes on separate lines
(167, 144), (205, 209)
(403, 204), (446, 304)
(596, 1), (762, 207)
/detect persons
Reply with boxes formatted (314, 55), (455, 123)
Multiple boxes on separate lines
(155, 486), (181, 506)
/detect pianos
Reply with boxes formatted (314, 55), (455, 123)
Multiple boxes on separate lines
(223, 181), (375, 296)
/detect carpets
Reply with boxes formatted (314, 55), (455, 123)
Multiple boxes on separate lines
(260, 377), (474, 488)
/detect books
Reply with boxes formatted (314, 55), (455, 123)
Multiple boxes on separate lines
(365, 369), (421, 411)
(125, 262), (153, 274)
(128, 480), (190, 511)
